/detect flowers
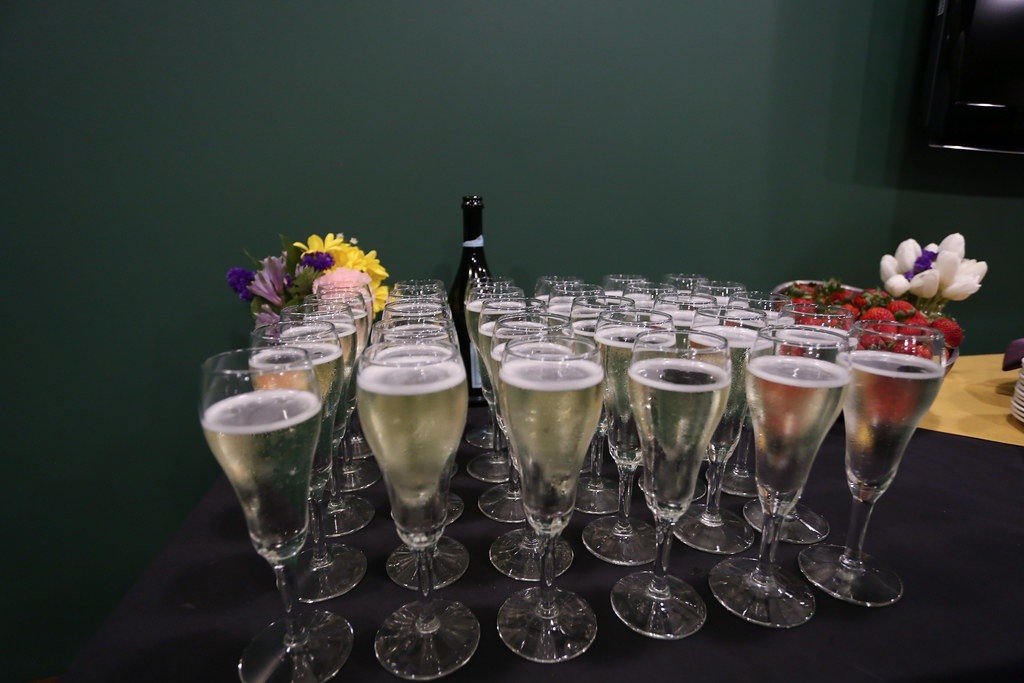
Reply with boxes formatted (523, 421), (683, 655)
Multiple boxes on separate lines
(879, 234), (989, 307)
(228, 233), (392, 339)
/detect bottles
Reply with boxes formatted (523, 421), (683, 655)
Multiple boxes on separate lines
(448, 196), (496, 394)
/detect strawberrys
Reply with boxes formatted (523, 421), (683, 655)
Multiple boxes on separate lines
(773, 277), (963, 359)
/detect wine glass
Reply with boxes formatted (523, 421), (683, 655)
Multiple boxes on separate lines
(198, 273), (948, 683)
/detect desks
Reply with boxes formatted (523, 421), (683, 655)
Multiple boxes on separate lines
(55, 352), (1024, 683)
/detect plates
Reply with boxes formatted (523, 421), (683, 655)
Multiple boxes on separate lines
(1011, 360), (1024, 422)
